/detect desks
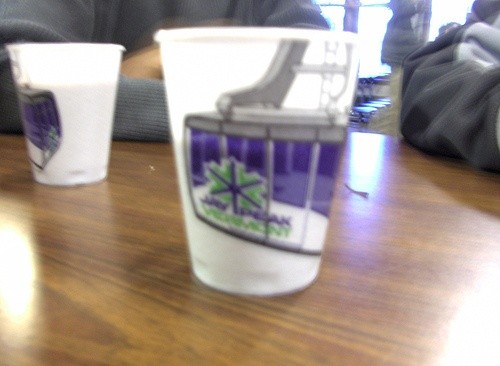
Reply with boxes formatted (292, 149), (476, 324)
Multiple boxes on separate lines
(0, 131), (500, 366)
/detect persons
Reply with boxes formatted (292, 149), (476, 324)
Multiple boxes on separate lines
(0, 0), (330, 141)
(381, 0), (428, 135)
(400, 0), (500, 174)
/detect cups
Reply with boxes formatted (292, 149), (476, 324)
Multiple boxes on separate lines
(153, 26), (362, 296)
(4, 41), (126, 187)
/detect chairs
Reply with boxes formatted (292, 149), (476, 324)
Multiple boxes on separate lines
(351, 74), (391, 131)
